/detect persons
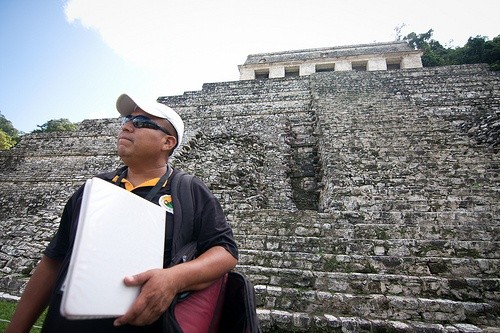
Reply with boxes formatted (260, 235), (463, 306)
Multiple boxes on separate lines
(6, 93), (238, 333)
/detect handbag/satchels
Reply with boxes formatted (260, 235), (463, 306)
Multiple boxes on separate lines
(162, 241), (228, 333)
(217, 271), (261, 333)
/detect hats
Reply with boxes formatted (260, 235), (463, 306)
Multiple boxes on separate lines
(116, 93), (184, 155)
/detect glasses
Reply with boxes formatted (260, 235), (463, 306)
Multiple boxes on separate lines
(122, 115), (177, 148)
